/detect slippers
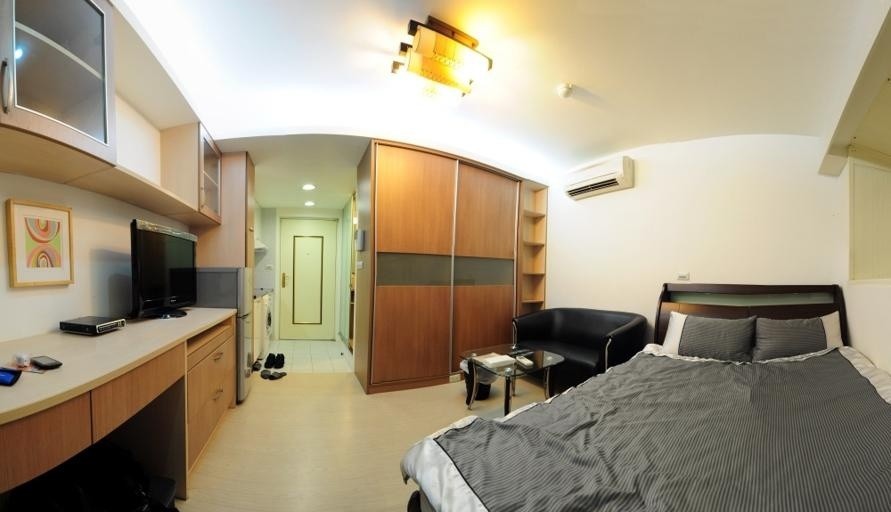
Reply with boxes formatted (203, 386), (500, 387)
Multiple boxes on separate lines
(269, 372), (286, 380)
(261, 370), (271, 379)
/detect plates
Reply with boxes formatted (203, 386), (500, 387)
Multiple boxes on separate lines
(1, 368), (23, 387)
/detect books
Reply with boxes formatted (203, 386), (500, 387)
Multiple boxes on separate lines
(473, 351), (517, 368)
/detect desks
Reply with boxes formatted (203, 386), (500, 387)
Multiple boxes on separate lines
(455, 340), (567, 421)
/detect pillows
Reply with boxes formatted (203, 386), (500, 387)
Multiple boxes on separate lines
(661, 309), (759, 364)
(746, 309), (847, 365)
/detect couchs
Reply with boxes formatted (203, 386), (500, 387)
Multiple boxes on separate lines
(511, 306), (649, 399)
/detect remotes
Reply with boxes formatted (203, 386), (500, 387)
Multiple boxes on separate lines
(517, 355), (534, 366)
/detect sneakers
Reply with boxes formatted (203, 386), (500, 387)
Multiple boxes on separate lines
(265, 354), (275, 368)
(275, 354), (284, 368)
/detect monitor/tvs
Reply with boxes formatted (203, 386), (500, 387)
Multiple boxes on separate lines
(129, 218), (197, 318)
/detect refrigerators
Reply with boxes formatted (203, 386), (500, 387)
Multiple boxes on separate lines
(196, 266), (254, 406)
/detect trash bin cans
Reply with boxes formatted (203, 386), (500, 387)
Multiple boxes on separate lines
(463, 357), (494, 400)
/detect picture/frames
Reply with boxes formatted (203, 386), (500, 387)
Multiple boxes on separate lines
(4, 195), (77, 290)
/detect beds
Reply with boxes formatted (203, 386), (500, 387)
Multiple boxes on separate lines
(400, 281), (891, 511)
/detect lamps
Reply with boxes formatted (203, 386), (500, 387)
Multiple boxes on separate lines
(386, 13), (494, 104)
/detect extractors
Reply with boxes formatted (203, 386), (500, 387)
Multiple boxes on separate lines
(254, 201), (269, 253)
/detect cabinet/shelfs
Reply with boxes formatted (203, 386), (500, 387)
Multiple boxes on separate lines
(350, 138), (520, 396)
(1, 1), (224, 228)
(515, 177), (549, 319)
(0, 332), (186, 503)
(111, 301), (239, 486)
(252, 297), (263, 365)
(192, 148), (256, 269)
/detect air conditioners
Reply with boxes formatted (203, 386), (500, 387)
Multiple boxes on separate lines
(563, 152), (636, 201)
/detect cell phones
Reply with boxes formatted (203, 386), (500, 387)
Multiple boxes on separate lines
(30, 356), (61, 370)
